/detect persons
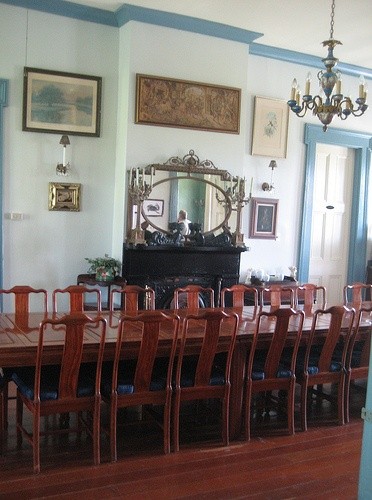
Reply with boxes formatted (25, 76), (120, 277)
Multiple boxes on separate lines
(178, 210), (192, 240)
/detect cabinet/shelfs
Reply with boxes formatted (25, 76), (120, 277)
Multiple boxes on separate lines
(121, 242), (246, 310)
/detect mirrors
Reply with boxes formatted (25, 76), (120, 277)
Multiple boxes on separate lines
(126, 149), (243, 244)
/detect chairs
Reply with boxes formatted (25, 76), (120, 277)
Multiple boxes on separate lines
(0, 283), (372, 473)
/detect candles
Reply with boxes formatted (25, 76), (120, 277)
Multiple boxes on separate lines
(230, 175), (253, 198)
(129, 166), (153, 188)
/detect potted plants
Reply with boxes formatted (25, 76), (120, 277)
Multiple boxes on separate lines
(85, 253), (122, 281)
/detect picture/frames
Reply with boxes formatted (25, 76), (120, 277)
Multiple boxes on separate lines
(21, 66), (102, 138)
(135, 73), (241, 135)
(250, 196), (280, 240)
(251, 95), (289, 159)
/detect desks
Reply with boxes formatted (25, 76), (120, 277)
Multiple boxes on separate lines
(238, 276), (317, 307)
(77, 274), (124, 311)
(0, 303), (372, 439)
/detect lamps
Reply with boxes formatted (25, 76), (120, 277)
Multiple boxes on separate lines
(56, 132), (70, 176)
(287, 0), (368, 132)
(262, 160), (278, 192)
